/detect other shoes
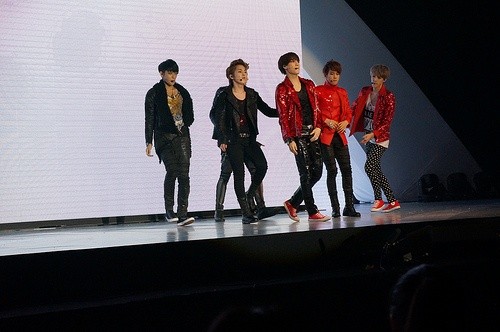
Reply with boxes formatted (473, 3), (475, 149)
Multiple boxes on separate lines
(308, 211), (331, 222)
(165, 211), (195, 226)
(283, 199), (300, 222)
(371, 200), (401, 212)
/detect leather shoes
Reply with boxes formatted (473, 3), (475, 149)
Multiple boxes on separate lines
(332, 207), (361, 217)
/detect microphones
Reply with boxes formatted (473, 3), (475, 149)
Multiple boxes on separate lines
(331, 80), (332, 82)
(371, 82), (373, 84)
(240, 79), (242, 81)
(295, 68), (297, 69)
(171, 80), (173, 82)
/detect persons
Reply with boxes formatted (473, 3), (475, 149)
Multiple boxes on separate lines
(145, 59), (195, 227)
(209, 67), (278, 222)
(275, 52), (330, 223)
(349, 64), (400, 212)
(316, 59), (361, 218)
(219, 59), (280, 223)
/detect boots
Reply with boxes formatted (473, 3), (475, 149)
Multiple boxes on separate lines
(215, 180), (278, 228)
